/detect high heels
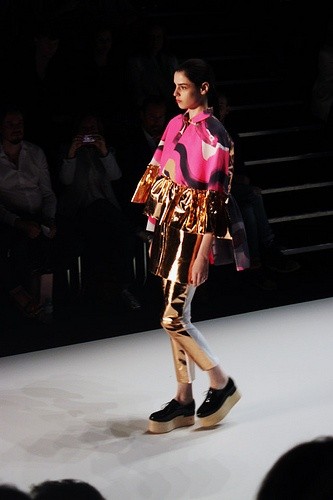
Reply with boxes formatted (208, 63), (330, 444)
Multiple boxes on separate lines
(197, 377), (240, 427)
(148, 399), (195, 433)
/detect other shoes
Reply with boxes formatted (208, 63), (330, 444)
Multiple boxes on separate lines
(40, 305), (54, 328)
(20, 303), (45, 319)
(122, 289), (141, 311)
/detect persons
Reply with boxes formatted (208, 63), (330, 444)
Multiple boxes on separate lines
(0, 104), (58, 318)
(311, 41), (333, 130)
(215, 92), (301, 274)
(47, 110), (125, 300)
(129, 54), (242, 433)
(109, 95), (171, 222)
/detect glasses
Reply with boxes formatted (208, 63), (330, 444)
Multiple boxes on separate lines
(5, 122), (24, 130)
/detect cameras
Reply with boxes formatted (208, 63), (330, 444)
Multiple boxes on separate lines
(81, 134), (100, 143)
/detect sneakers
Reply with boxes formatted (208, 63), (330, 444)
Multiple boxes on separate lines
(287, 258), (301, 272)
